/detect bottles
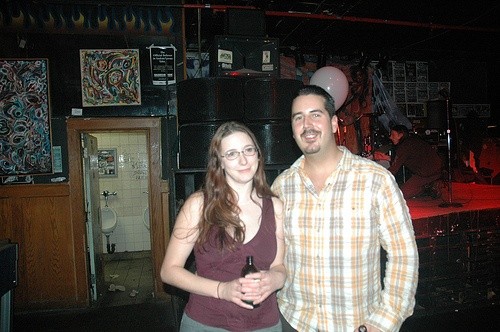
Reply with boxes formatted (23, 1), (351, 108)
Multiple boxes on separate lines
(241, 256), (260, 308)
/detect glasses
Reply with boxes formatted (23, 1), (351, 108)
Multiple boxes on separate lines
(221, 148), (257, 160)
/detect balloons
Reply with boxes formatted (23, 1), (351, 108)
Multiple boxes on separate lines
(311, 66), (350, 112)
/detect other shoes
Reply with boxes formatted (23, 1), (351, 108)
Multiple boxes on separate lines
(432, 182), (441, 200)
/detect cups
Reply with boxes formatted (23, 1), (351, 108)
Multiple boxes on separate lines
(107, 243), (115, 253)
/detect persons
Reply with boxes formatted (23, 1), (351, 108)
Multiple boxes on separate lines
(271, 85), (419, 332)
(387, 125), (445, 200)
(188, 59), (210, 79)
(160, 121), (287, 332)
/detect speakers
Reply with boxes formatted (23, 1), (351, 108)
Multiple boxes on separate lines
(175, 34), (303, 215)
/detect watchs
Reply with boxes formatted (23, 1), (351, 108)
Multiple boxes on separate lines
(358, 323), (368, 332)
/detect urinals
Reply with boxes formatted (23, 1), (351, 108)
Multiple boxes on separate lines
(101, 206), (118, 235)
(143, 205), (150, 231)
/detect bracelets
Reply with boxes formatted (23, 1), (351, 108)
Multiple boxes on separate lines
(216, 280), (222, 299)
